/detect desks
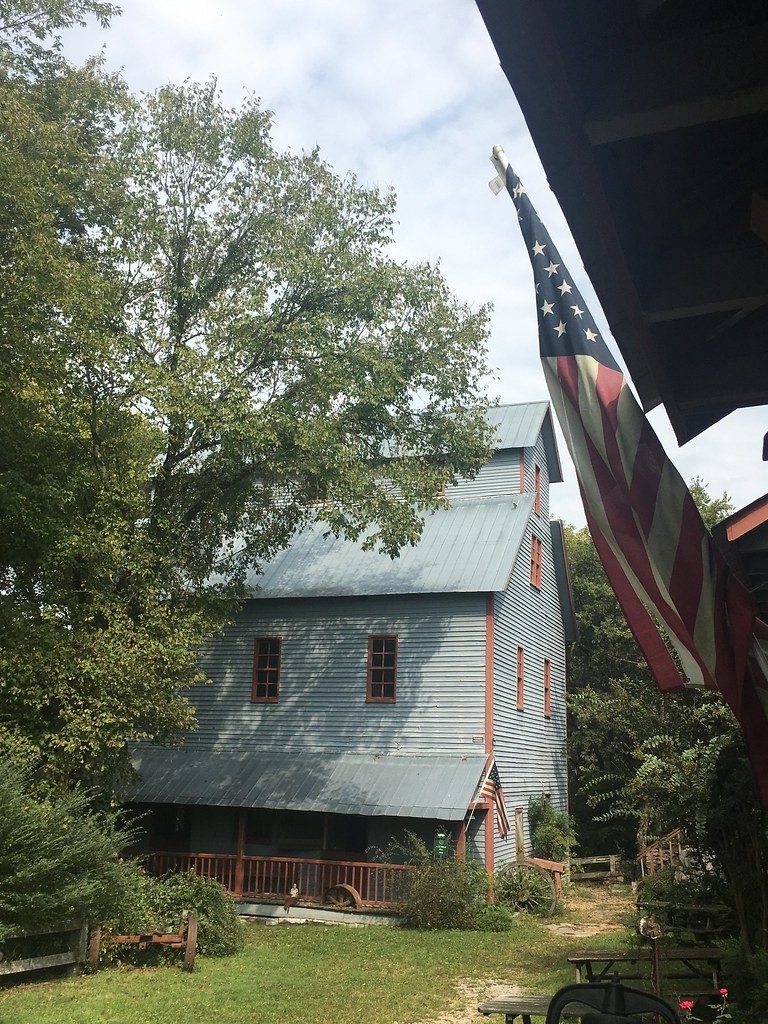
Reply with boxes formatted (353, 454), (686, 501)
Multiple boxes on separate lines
(567, 945), (723, 1013)
(639, 900), (734, 946)
(474, 995), (702, 1024)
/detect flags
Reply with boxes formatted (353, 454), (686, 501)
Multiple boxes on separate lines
(504, 163), (768, 807)
(480, 758), (511, 842)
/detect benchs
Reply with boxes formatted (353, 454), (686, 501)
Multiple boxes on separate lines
(624, 921), (734, 947)
(586, 971), (727, 991)
(651, 989), (729, 1015)
